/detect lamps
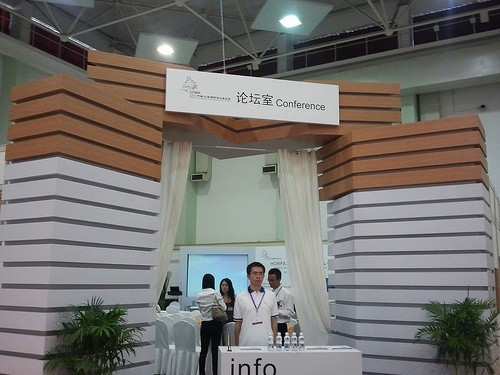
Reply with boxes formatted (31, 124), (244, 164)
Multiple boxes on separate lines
(249, 0), (334, 36)
(134, 32), (200, 64)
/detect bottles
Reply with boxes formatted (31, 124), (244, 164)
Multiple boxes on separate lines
(276, 332), (282, 351)
(284, 332), (290, 350)
(298, 332), (305, 351)
(268, 333), (274, 351)
(291, 332), (297, 351)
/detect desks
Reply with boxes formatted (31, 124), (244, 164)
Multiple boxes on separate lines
(218, 345), (364, 375)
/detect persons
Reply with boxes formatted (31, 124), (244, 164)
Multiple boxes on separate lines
(267, 268), (295, 346)
(220, 278), (236, 346)
(233, 261), (280, 346)
(197, 273), (227, 375)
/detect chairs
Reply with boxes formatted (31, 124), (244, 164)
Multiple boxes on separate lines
(155, 301), (236, 375)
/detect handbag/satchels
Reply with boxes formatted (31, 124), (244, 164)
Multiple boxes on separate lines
(212, 306), (228, 323)
